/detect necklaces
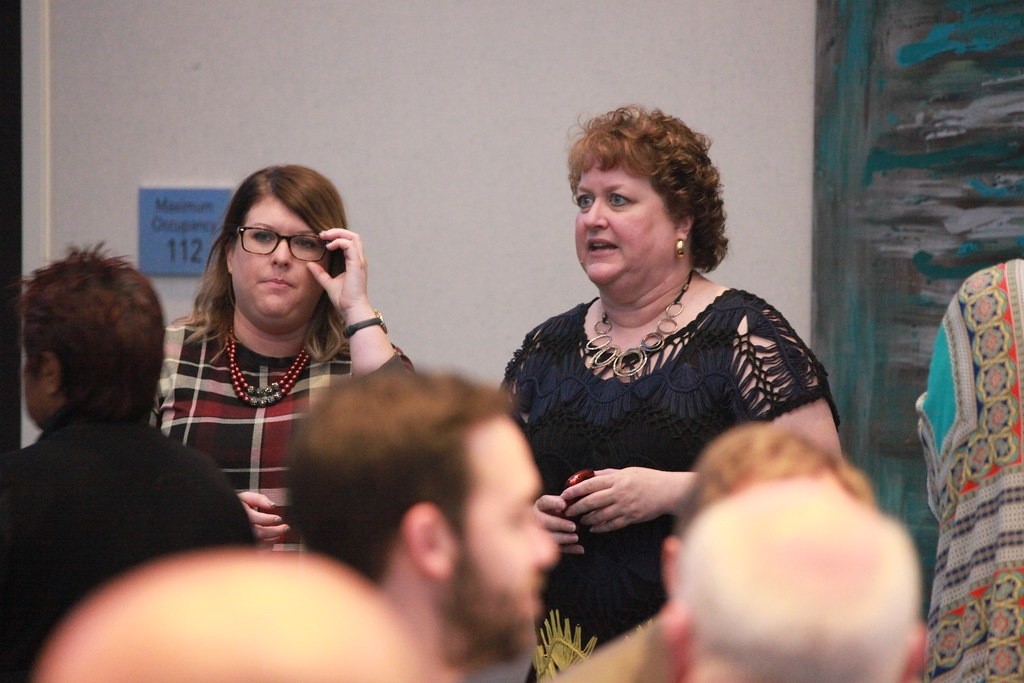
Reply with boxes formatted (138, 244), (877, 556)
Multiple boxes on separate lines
(224, 325), (310, 407)
(585, 270), (692, 377)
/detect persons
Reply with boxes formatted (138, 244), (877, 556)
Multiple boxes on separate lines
(550, 421), (877, 683)
(34, 546), (429, 683)
(911, 258), (1024, 683)
(146, 165), (416, 560)
(14, 240), (258, 682)
(662, 480), (929, 683)
(282, 373), (557, 683)
(497, 106), (846, 683)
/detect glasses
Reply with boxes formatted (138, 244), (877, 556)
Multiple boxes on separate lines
(237, 226), (328, 262)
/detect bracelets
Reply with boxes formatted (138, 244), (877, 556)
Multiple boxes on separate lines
(343, 309), (388, 338)
